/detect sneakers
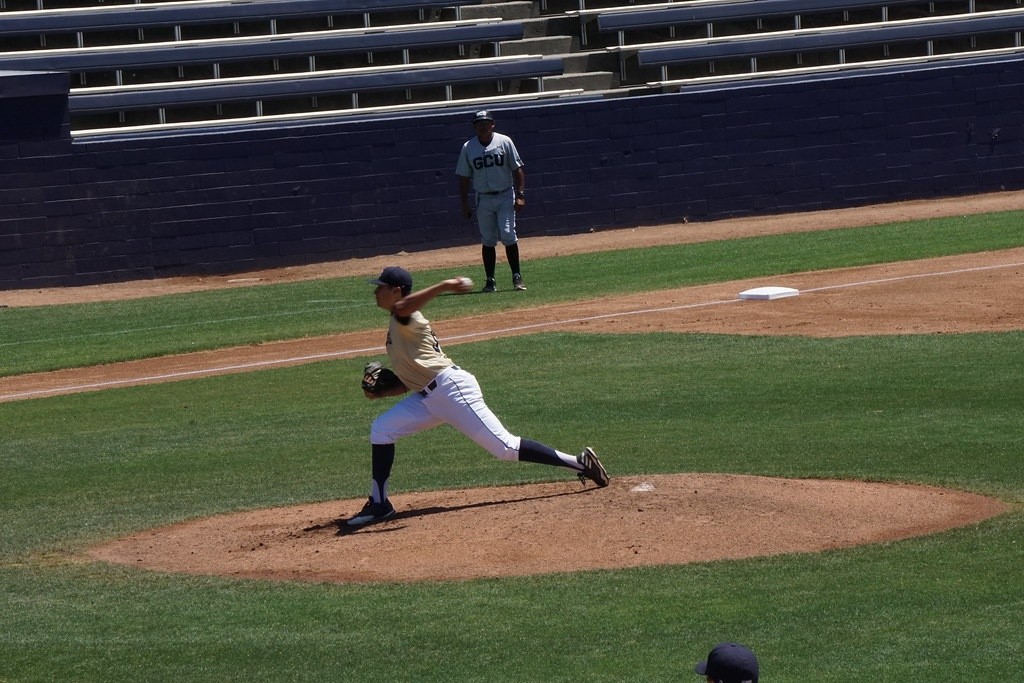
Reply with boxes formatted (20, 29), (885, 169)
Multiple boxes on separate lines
(346, 496), (395, 528)
(577, 447), (609, 487)
(512, 273), (526, 291)
(482, 278), (497, 292)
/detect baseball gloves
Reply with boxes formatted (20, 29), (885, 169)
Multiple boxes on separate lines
(361, 361), (405, 396)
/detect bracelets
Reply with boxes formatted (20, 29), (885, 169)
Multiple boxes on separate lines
(517, 191), (525, 199)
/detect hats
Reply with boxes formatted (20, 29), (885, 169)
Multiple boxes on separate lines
(368, 266), (412, 287)
(472, 110), (493, 123)
(694, 642), (759, 683)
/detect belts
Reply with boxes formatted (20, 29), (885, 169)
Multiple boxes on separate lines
(479, 187), (512, 195)
(419, 379), (437, 397)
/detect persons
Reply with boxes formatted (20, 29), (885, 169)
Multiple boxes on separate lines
(695, 643), (759, 683)
(455, 110), (527, 292)
(344, 266), (609, 530)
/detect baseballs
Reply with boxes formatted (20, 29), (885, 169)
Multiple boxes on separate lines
(459, 277), (473, 285)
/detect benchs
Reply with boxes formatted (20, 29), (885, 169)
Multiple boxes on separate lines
(0, 0), (1024, 129)
